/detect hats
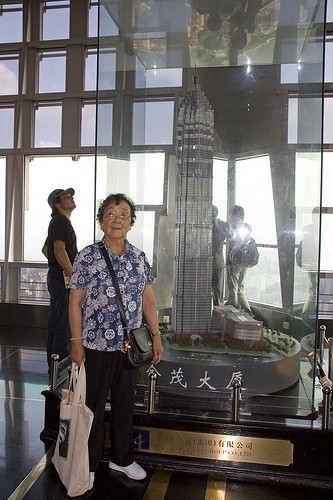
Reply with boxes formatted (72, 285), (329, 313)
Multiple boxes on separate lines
(48, 187), (75, 211)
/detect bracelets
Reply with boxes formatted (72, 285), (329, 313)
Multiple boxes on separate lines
(151, 332), (160, 337)
(69, 337), (85, 340)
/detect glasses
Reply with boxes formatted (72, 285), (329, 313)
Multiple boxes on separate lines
(104, 211), (132, 219)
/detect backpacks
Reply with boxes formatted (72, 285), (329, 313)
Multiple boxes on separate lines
(296, 233), (316, 270)
(237, 223), (259, 268)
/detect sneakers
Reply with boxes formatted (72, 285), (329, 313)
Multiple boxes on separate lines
(108, 460), (148, 480)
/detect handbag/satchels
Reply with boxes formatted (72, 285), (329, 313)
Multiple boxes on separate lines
(50, 361), (94, 497)
(125, 325), (154, 369)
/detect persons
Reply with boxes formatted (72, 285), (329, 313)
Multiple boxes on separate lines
(223, 205), (253, 318)
(42, 187), (79, 377)
(211, 205), (230, 306)
(68, 193), (165, 491)
